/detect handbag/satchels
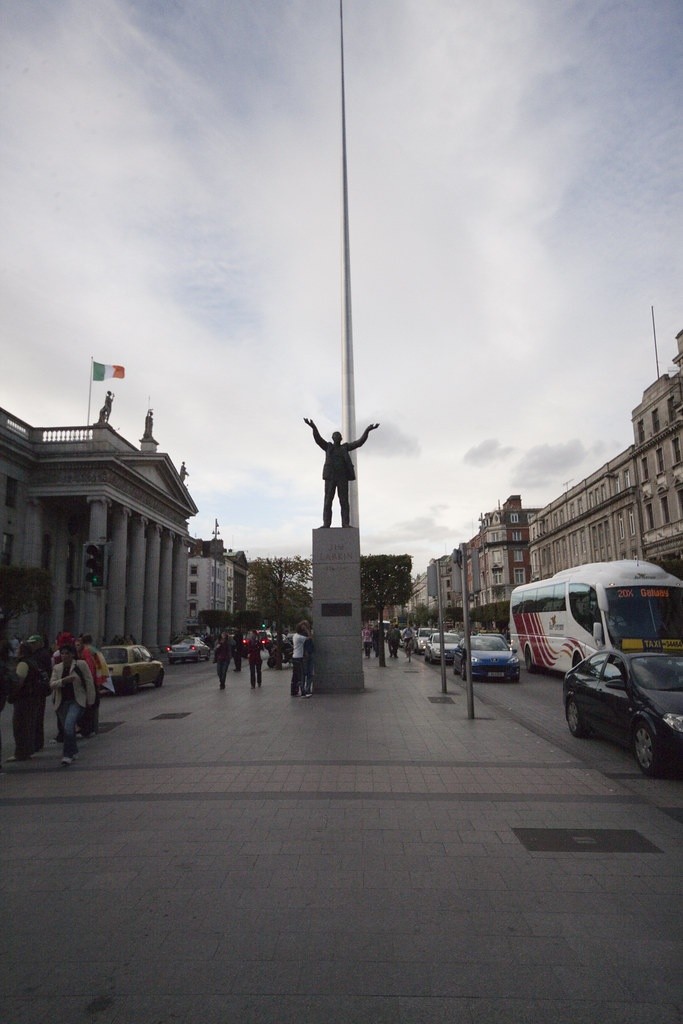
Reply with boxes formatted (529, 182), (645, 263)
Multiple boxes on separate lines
(37, 670), (52, 697)
(260, 649), (270, 661)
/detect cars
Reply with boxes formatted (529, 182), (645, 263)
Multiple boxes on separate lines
(477, 632), (511, 648)
(243, 630), (271, 649)
(383, 621), (405, 646)
(452, 635), (520, 682)
(166, 636), (210, 664)
(563, 650), (683, 779)
(425, 633), (461, 663)
(101, 645), (165, 696)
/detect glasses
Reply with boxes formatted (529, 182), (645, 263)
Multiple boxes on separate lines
(20, 641), (27, 647)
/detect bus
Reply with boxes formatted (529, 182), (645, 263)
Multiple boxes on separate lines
(509, 558), (683, 675)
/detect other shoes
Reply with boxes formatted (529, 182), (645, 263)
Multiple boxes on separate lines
(300, 693), (312, 698)
(220, 684), (226, 690)
(292, 693), (301, 697)
(233, 668), (241, 672)
(251, 683), (261, 690)
(62, 753), (79, 764)
(7, 756), (31, 761)
(77, 732), (96, 739)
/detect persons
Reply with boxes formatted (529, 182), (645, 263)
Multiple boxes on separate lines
(213, 630), (236, 689)
(180, 461), (189, 484)
(170, 631), (212, 650)
(363, 624), (373, 659)
(371, 625), (380, 658)
(110, 634), (137, 645)
(303, 417), (380, 529)
(143, 411), (154, 438)
(98, 391), (115, 423)
(26, 634), (53, 752)
(48, 631), (109, 744)
(402, 625), (415, 656)
(6, 639), (40, 762)
(0, 630), (14, 771)
(9, 634), (19, 656)
(233, 629), (243, 673)
(246, 629), (265, 689)
(291, 620), (315, 698)
(49, 642), (96, 766)
(386, 624), (403, 659)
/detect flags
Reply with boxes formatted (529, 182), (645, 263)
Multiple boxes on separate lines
(92, 361), (125, 381)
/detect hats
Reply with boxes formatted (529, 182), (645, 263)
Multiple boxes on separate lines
(26, 635), (43, 643)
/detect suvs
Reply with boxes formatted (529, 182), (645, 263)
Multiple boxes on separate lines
(414, 627), (438, 653)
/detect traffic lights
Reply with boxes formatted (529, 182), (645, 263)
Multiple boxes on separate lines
(85, 543), (105, 587)
